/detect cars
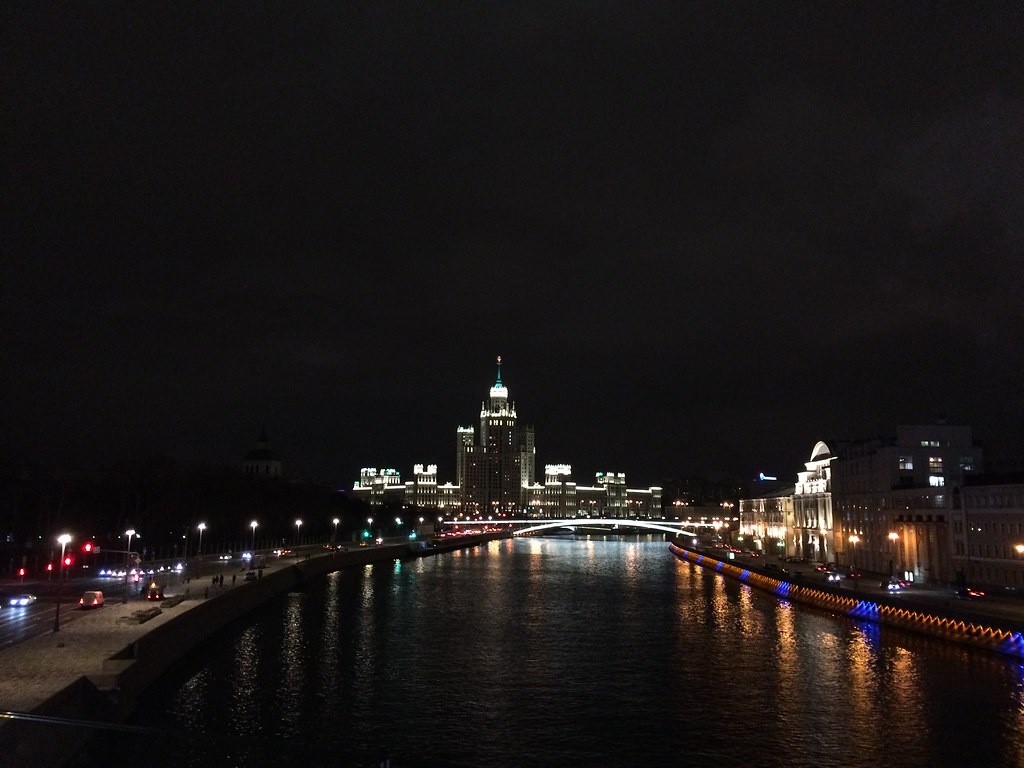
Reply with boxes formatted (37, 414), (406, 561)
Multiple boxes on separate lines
(322, 543), (336, 551)
(376, 541), (383, 545)
(99, 561), (187, 577)
(435, 528), (503, 538)
(220, 554), (232, 560)
(763, 556), (984, 600)
(274, 548), (283, 553)
(148, 589), (163, 601)
(79, 591), (105, 608)
(360, 541), (367, 547)
(285, 548), (294, 554)
(142, 583), (160, 592)
(243, 572), (257, 581)
(711, 539), (760, 557)
(10, 594), (38, 606)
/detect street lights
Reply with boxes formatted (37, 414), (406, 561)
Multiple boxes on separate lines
(53, 534), (71, 631)
(251, 522), (257, 569)
(849, 536), (860, 586)
(368, 518), (372, 546)
(197, 523), (205, 578)
(296, 520), (301, 563)
(420, 518), (423, 541)
(333, 519), (339, 550)
(123, 530), (135, 603)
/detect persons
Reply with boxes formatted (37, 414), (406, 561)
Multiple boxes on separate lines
(211, 575), (223, 587)
(205, 588), (208, 598)
(232, 574), (236, 583)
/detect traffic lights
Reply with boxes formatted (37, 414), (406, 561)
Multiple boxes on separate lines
(84, 544), (92, 552)
